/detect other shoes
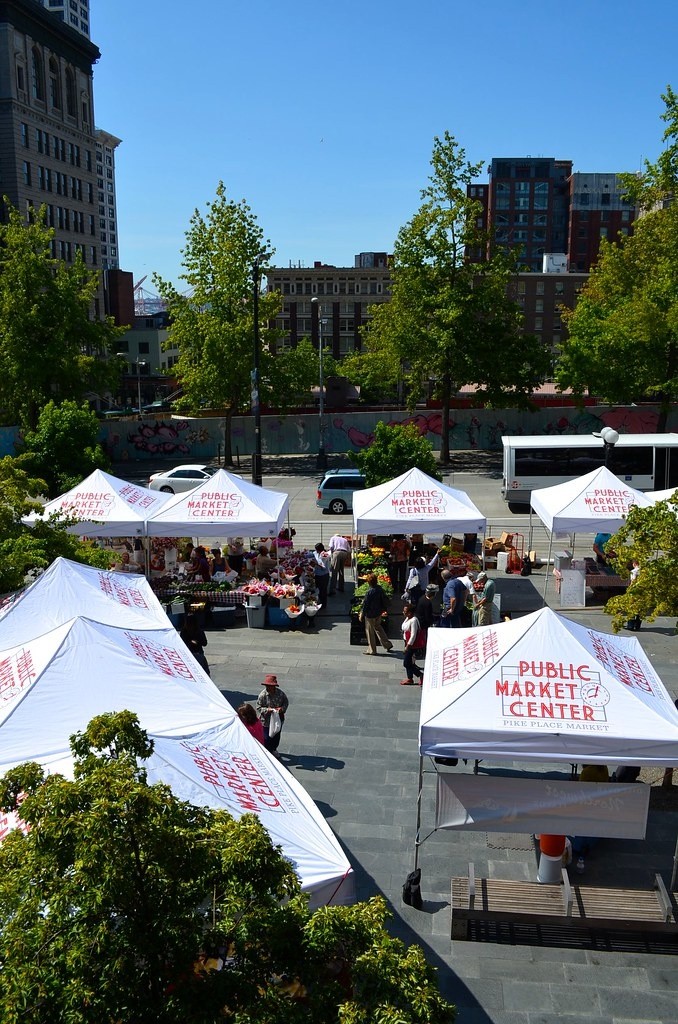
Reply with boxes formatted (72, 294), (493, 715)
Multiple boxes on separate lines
(400, 673), (423, 685)
(363, 645), (393, 655)
(318, 601), (328, 609)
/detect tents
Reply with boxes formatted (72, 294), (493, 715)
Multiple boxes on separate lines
(401, 604), (678, 915)
(1, 554), (357, 938)
(18, 469), (173, 590)
(146, 465), (294, 596)
(525, 464), (658, 608)
(352, 464), (487, 610)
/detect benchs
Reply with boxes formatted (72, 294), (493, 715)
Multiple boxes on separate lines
(450, 860), (678, 932)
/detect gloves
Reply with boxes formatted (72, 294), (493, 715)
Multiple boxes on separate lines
(268, 707), (282, 713)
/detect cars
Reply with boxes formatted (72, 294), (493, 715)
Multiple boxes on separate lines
(96, 400), (176, 416)
(149, 463), (241, 495)
(317, 466), (373, 513)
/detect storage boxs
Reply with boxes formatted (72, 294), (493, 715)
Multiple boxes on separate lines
(483, 529), (536, 576)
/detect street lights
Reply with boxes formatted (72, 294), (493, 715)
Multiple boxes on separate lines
(598, 426), (620, 469)
(115, 351), (146, 414)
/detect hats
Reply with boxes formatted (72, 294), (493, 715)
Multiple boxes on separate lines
(476, 572), (485, 583)
(261, 674), (280, 687)
(425, 583), (439, 592)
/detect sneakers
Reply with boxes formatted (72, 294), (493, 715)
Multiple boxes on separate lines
(272, 750), (280, 758)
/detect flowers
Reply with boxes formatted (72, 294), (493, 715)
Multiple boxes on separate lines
(83, 533), (328, 618)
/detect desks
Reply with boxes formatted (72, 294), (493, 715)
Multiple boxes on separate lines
(587, 567), (630, 587)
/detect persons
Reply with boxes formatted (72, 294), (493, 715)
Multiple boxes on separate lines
(397, 603), (424, 686)
(326, 534), (352, 597)
(473, 572), (496, 627)
(311, 542), (331, 608)
(357, 574), (393, 655)
(622, 558), (645, 630)
(80, 526), (298, 583)
(578, 763), (646, 784)
(592, 532), (615, 569)
(388, 534), (480, 658)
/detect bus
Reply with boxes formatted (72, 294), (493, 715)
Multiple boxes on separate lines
(500, 426), (678, 507)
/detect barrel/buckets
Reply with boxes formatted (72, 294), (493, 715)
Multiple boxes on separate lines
(245, 602), (267, 628)
(553, 549), (573, 572)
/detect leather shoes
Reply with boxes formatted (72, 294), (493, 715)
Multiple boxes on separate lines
(327, 588), (345, 595)
